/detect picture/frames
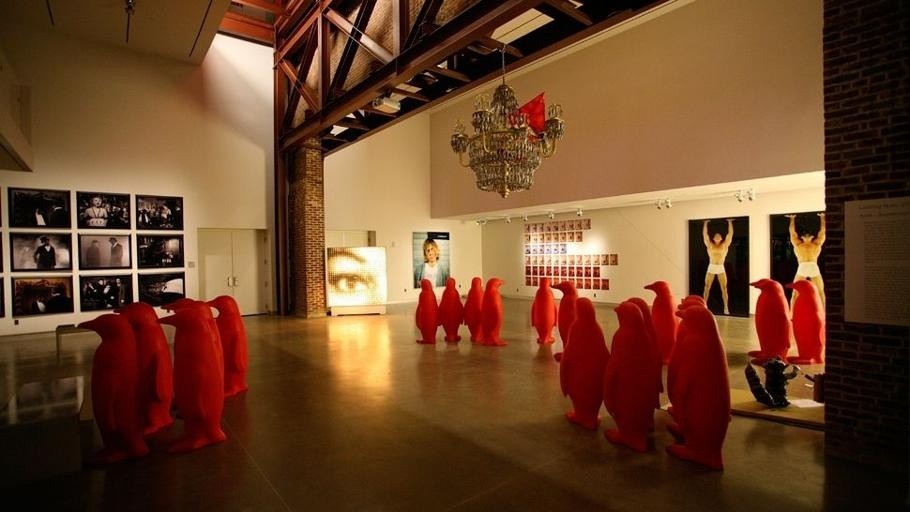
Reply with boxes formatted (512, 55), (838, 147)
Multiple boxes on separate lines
(0, 187), (185, 318)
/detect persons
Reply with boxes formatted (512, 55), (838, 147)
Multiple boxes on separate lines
(8, 187), (185, 316)
(785, 213), (826, 320)
(703, 219), (734, 315)
(415, 238), (446, 288)
(325, 247), (386, 307)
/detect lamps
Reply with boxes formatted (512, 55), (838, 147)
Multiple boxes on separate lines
(451, 43), (566, 199)
(476, 188), (755, 226)
